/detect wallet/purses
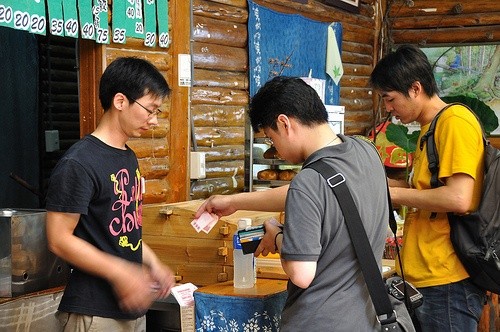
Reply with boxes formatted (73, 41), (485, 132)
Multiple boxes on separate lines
(238, 225), (284, 254)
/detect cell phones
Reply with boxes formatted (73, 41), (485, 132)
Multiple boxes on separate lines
(394, 281), (423, 302)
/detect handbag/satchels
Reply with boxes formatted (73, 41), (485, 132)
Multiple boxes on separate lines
(376, 289), (416, 332)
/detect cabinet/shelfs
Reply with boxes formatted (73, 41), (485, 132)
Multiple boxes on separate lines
(253, 137), (304, 186)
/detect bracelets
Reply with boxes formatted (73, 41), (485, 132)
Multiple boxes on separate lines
(275, 231), (283, 251)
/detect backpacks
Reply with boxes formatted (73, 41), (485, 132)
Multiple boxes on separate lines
(428, 103), (500, 294)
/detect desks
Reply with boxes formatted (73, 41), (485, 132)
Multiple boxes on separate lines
(193, 266), (396, 332)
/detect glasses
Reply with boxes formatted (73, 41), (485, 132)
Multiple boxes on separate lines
(264, 113), (289, 145)
(113, 91), (162, 117)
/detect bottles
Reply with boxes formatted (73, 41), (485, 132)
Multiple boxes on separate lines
(233, 218), (257, 288)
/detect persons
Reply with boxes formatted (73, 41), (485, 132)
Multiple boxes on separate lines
(371, 46), (488, 332)
(195, 75), (389, 332)
(46, 57), (175, 332)
